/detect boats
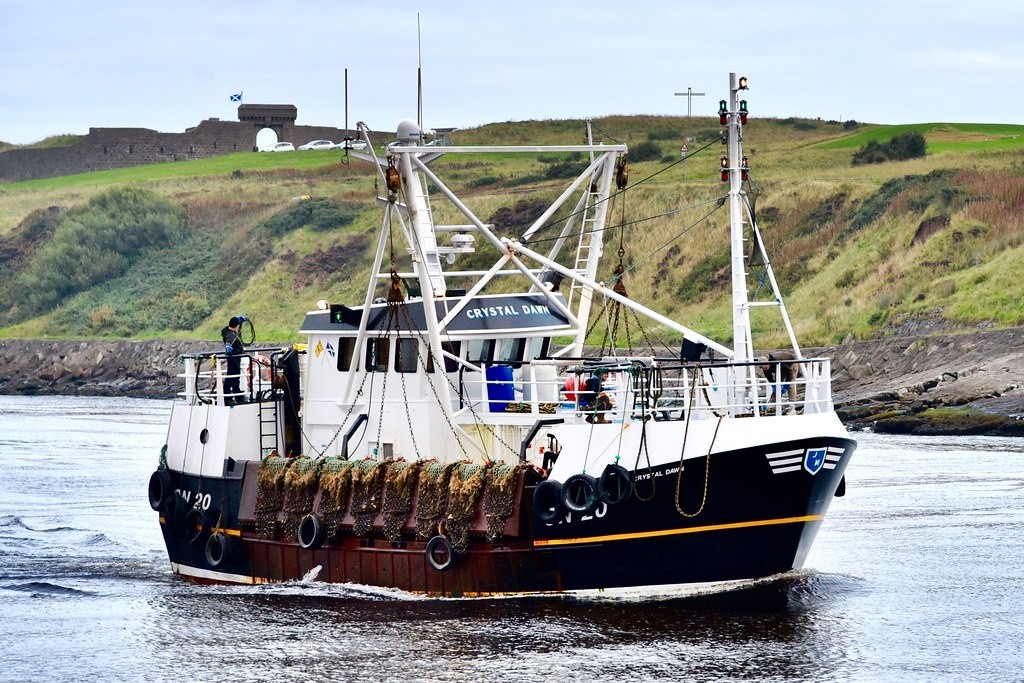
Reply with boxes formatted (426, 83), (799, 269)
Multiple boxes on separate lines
(148, 73), (859, 591)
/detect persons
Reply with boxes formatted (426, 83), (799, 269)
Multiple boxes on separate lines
(579, 368), (613, 424)
(758, 350), (799, 415)
(221, 317), (245, 394)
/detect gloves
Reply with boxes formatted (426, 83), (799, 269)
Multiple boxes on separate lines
(226, 345), (233, 352)
(238, 316), (246, 322)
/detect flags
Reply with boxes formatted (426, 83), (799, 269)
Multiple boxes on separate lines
(230, 94), (241, 101)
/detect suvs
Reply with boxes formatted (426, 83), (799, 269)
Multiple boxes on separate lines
(329, 139), (366, 150)
(298, 140), (335, 151)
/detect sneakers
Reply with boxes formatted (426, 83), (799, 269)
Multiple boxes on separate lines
(788, 410), (796, 415)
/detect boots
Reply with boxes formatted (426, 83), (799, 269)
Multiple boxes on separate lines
(586, 416), (597, 424)
(598, 414), (612, 423)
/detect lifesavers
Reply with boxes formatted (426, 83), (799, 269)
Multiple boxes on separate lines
(597, 465), (633, 504)
(532, 479), (567, 523)
(163, 495), (186, 531)
(425, 535), (462, 572)
(297, 514), (326, 551)
(148, 470), (169, 510)
(247, 355), (272, 387)
(203, 532), (233, 567)
(561, 474), (601, 513)
(182, 508), (208, 545)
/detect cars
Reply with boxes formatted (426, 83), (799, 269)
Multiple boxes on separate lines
(261, 142), (295, 152)
